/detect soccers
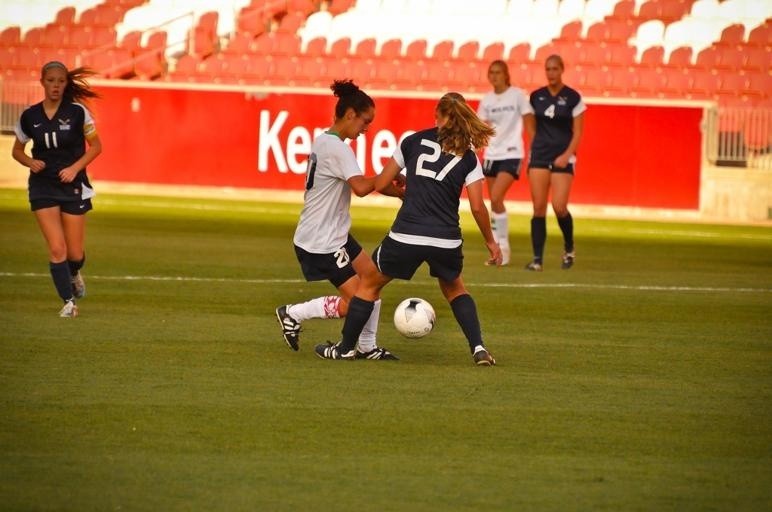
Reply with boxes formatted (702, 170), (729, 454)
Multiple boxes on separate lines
(394, 298), (436, 338)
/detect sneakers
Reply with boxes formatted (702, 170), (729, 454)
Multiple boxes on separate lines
(561, 249), (575, 269)
(59, 299), (79, 318)
(70, 270), (85, 299)
(274, 304), (302, 351)
(525, 261), (543, 271)
(355, 345), (400, 361)
(473, 349), (496, 366)
(500, 245), (511, 265)
(313, 340), (356, 360)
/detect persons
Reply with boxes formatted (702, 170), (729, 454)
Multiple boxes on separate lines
(477, 59), (536, 268)
(10, 60), (104, 317)
(314, 92), (504, 368)
(524, 54), (588, 271)
(275, 78), (406, 361)
